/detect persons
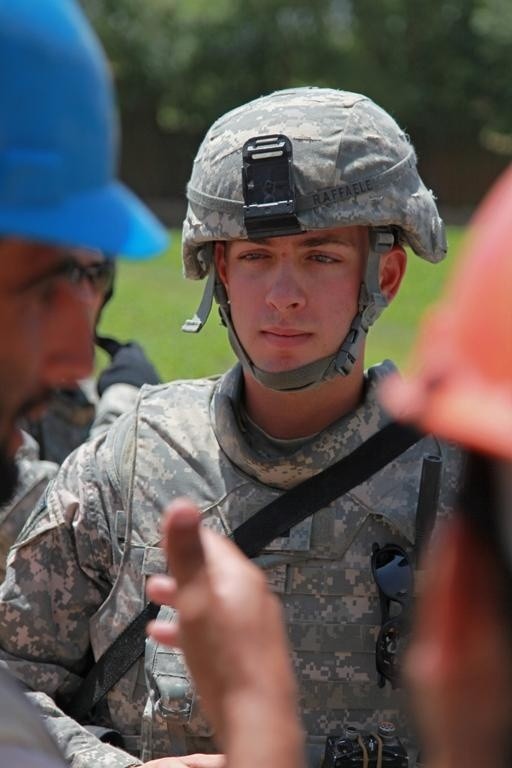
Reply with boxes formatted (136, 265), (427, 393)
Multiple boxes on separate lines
(1, 1), (318, 768)
(374, 166), (512, 768)
(1, 83), (482, 767)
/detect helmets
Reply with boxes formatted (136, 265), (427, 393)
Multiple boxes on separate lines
(0, 0), (171, 259)
(381, 161), (512, 457)
(183, 85), (446, 281)
